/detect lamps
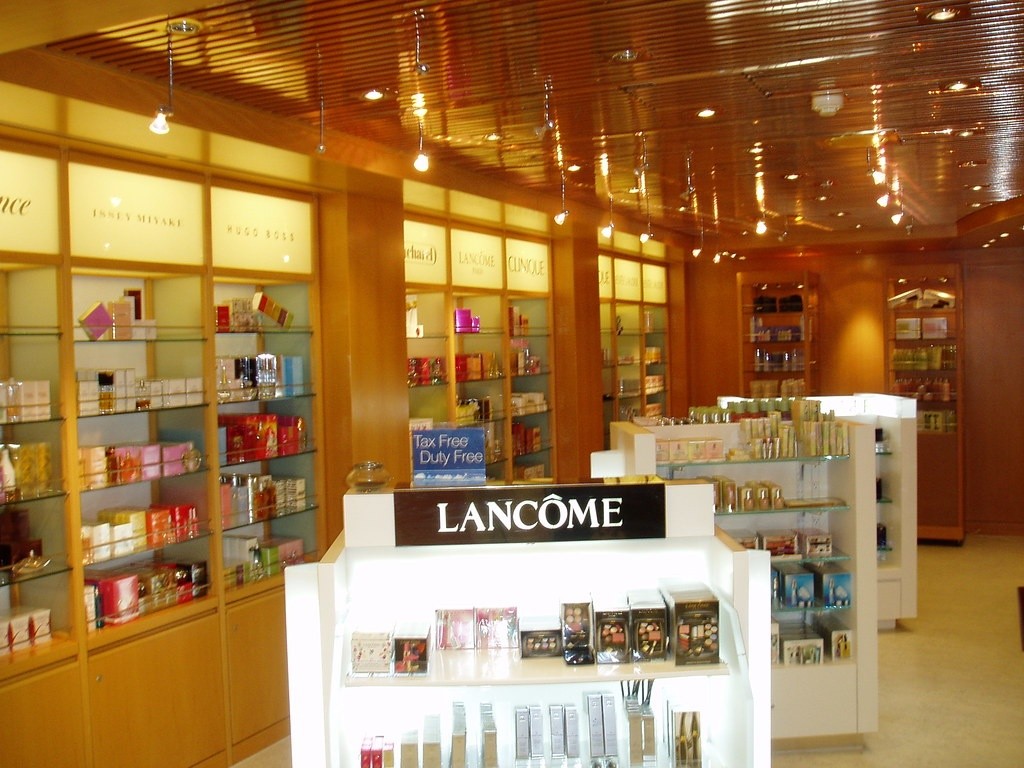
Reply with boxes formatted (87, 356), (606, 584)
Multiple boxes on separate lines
(555, 175), (569, 225)
(602, 197), (615, 237)
(149, 27), (173, 135)
(413, 110), (428, 172)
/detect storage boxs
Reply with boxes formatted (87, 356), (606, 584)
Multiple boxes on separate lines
(623, 698), (654, 766)
(697, 477), (781, 511)
(0, 289), (312, 649)
(733, 529), (852, 666)
(351, 607), (520, 674)
(360, 703), (498, 768)
(516, 692), (616, 761)
(406, 294), (547, 459)
(656, 440), (722, 462)
(521, 629), (563, 657)
(562, 588), (720, 666)
(664, 700), (703, 768)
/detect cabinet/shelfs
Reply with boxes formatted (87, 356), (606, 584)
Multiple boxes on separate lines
(281, 480), (779, 768)
(0, 79), (330, 768)
(883, 264), (965, 543)
(400, 177), (559, 486)
(597, 226), (672, 453)
(717, 393), (916, 630)
(737, 270), (819, 396)
(589, 416), (878, 738)
